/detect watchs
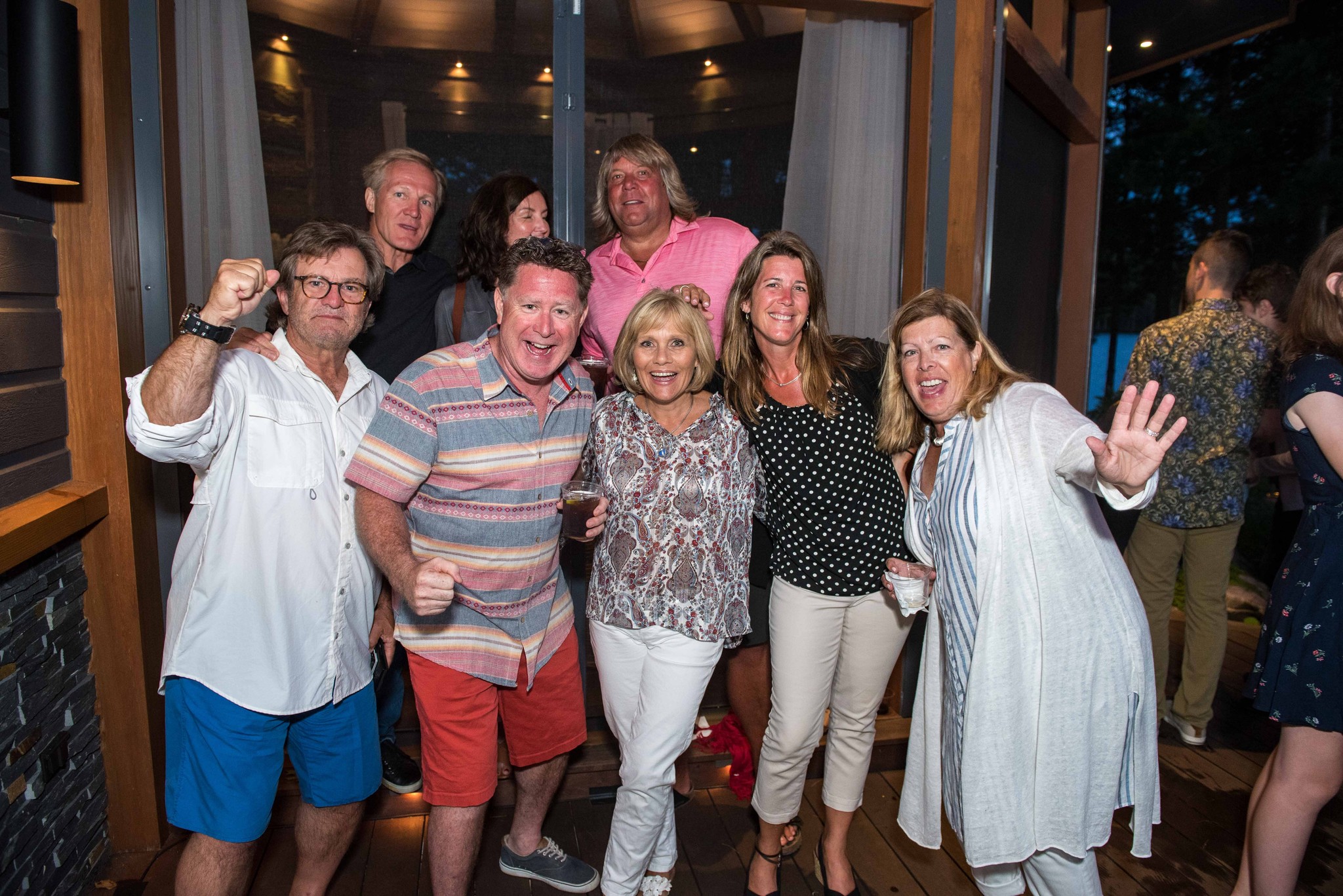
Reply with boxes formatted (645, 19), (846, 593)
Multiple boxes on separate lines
(176, 302), (237, 344)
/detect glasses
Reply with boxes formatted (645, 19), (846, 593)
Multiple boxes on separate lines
(293, 275), (369, 304)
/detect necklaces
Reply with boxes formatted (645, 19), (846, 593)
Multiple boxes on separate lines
(632, 258), (650, 264)
(931, 432), (947, 446)
(642, 393), (694, 457)
(760, 364), (802, 386)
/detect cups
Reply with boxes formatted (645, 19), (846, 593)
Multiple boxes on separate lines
(562, 481), (604, 536)
(574, 357), (608, 401)
(886, 564), (932, 614)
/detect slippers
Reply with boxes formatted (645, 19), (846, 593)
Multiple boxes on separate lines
(637, 866), (674, 896)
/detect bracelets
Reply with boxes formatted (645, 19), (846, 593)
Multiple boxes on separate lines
(905, 448), (915, 458)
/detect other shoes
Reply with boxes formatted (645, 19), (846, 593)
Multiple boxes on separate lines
(1162, 698), (1207, 744)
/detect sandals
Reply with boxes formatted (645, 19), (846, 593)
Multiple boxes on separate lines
(781, 817), (803, 855)
(493, 718), (511, 779)
(672, 780), (695, 809)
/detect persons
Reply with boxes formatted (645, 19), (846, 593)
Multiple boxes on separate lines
(1229, 230), (1343, 896)
(579, 287), (768, 896)
(670, 230), (918, 896)
(125, 222), (398, 896)
(342, 236), (610, 896)
(434, 173), (550, 349)
(575, 131), (759, 396)
(881, 288), (1187, 896)
(1116, 227), (1279, 746)
(225, 146), (453, 792)
(1233, 259), (1305, 590)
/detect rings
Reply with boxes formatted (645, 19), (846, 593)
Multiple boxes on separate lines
(1144, 426), (1160, 438)
(680, 285), (690, 293)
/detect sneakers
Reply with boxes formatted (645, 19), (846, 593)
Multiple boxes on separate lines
(375, 739), (422, 793)
(498, 834), (601, 893)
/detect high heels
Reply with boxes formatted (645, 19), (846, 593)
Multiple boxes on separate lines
(812, 832), (862, 896)
(743, 834), (783, 896)
